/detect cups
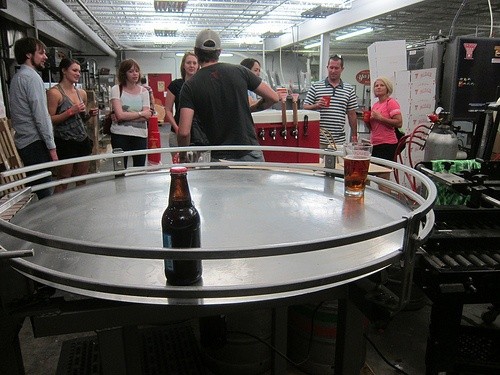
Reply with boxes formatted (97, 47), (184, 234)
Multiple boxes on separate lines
(343, 142), (373, 197)
(90, 109), (97, 117)
(277, 88), (286, 100)
(321, 96), (331, 107)
(364, 111), (371, 122)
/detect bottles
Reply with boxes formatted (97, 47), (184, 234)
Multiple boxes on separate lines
(79, 109), (91, 122)
(162, 167), (202, 285)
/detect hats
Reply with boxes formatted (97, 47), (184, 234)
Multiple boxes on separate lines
(195, 27), (222, 50)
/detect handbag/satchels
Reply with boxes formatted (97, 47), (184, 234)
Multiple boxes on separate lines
(394, 128), (406, 154)
(104, 83), (123, 135)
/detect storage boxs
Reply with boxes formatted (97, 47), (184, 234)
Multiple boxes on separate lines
(252, 109), (321, 162)
(367, 40), (436, 195)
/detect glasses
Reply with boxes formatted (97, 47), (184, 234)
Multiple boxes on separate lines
(330, 53), (343, 60)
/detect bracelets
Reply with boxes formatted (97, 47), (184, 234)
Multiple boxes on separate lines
(138, 112), (141, 119)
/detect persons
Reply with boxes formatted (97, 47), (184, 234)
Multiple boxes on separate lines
(9, 36), (59, 201)
(237, 58), (263, 108)
(164, 52), (201, 163)
(140, 77), (155, 116)
(362, 77), (402, 193)
(46, 57), (98, 193)
(109, 59), (152, 178)
(177, 29), (279, 162)
(302, 54), (359, 163)
